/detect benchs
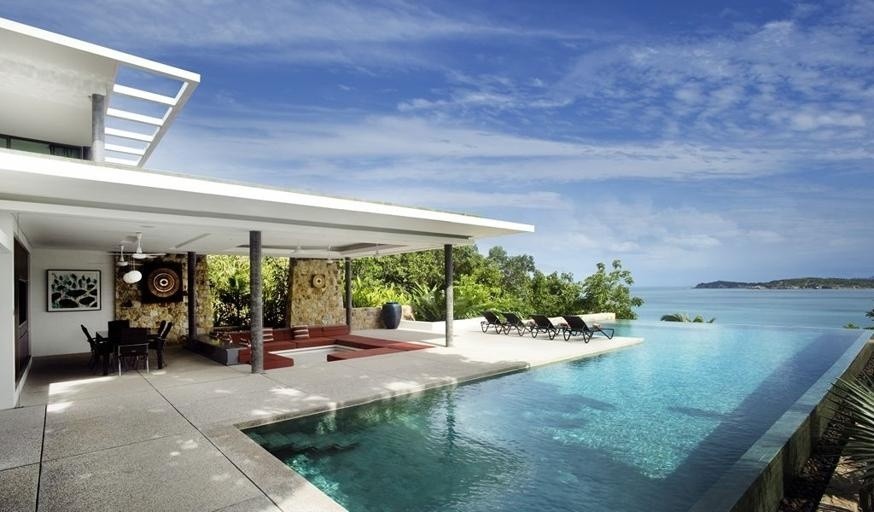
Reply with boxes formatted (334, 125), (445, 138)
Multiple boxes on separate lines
(327, 335), (434, 361)
(227, 325), (350, 371)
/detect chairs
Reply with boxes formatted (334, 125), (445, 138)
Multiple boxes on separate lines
(81, 319), (173, 377)
(480, 311), (615, 343)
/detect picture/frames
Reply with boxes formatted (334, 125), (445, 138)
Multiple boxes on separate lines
(45, 269), (101, 311)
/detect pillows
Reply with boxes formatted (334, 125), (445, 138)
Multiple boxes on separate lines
(209, 331), (233, 346)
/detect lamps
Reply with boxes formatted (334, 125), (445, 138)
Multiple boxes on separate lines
(123, 254), (142, 283)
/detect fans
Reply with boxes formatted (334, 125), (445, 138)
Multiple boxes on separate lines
(120, 232), (167, 260)
(105, 246), (142, 266)
